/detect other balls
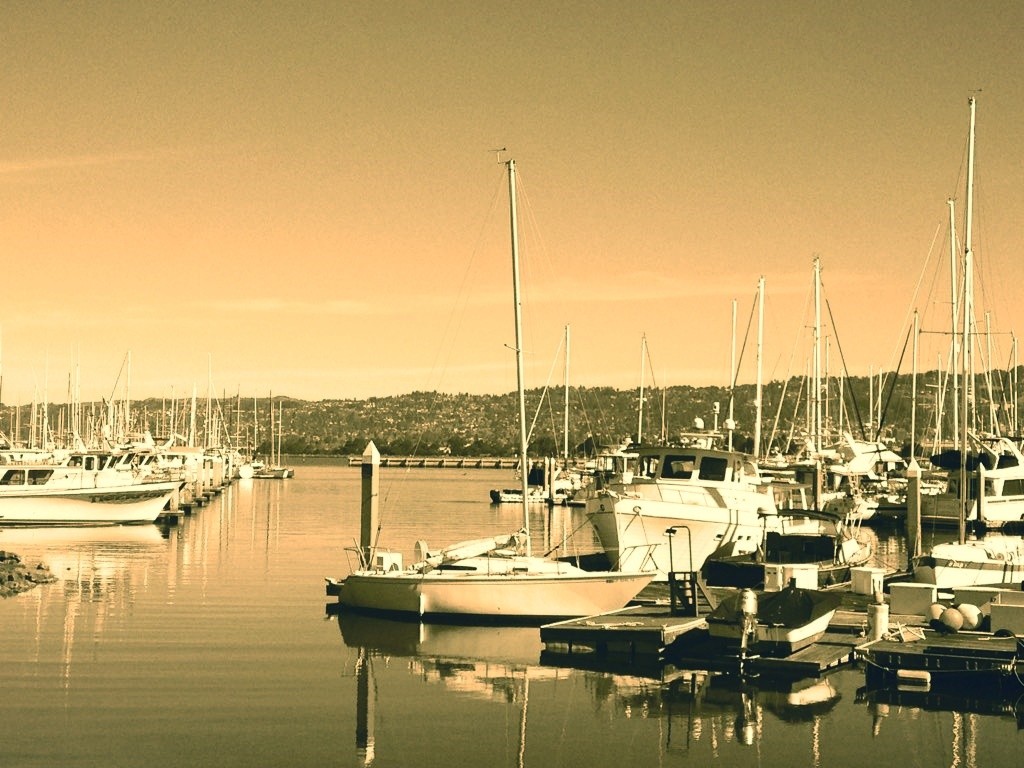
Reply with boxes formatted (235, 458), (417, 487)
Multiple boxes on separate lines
(957, 603), (983, 630)
(938, 608), (964, 633)
(923, 604), (948, 624)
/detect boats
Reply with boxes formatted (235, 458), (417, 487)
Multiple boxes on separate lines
(338, 92), (1024, 625)
(0, 350), (294, 529)
